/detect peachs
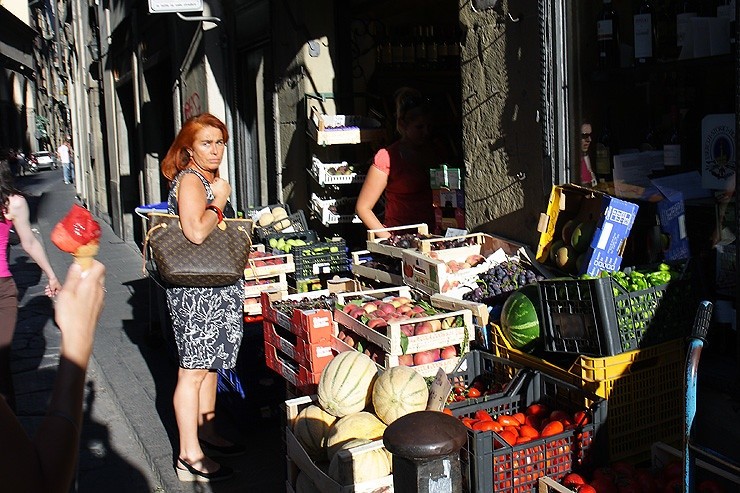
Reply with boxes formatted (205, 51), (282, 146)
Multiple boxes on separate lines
(423, 251), (489, 294)
(244, 251), (285, 304)
(337, 296), (461, 367)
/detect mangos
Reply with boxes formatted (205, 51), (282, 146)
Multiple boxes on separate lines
(327, 165), (354, 177)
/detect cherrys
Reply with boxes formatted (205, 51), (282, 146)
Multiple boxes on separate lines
(273, 292), (362, 316)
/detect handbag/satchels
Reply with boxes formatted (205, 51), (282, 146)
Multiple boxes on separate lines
(144, 170), (253, 287)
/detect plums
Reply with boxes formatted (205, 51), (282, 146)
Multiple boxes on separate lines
(380, 233), (471, 249)
(361, 256), (402, 273)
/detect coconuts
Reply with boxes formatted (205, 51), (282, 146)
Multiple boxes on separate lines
(548, 218), (596, 276)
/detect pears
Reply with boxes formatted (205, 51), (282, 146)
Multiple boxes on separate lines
(269, 237), (340, 275)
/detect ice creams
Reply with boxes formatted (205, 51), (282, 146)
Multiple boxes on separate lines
(51, 203), (101, 270)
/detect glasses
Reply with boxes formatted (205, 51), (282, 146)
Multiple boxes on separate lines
(580, 131), (596, 139)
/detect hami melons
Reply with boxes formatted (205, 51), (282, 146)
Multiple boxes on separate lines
(259, 207), (291, 231)
(292, 351), (429, 493)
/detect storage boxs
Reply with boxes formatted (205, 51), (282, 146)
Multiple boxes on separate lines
(243, 109), (740, 493)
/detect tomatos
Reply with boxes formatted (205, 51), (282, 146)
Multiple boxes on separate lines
(561, 459), (729, 493)
(441, 378), (593, 493)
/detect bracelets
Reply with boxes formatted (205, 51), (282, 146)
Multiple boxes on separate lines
(206, 203), (223, 223)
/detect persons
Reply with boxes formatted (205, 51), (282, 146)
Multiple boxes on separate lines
(0, 159), (62, 348)
(56, 139), (74, 184)
(7, 148), (39, 177)
(161, 114), (247, 481)
(578, 114), (597, 188)
(356, 87), (436, 238)
(53, 253), (107, 492)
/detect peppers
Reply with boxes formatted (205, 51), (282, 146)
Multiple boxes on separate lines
(557, 262), (680, 350)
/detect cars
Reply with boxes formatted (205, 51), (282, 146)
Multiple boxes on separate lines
(33, 151), (57, 170)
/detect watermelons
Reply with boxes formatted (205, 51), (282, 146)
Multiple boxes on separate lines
(500, 283), (546, 352)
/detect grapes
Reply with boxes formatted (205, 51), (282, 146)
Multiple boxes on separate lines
(463, 260), (546, 307)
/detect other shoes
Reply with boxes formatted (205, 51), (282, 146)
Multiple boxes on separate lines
(64, 181), (71, 185)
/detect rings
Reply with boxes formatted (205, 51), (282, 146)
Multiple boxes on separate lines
(102, 287), (108, 293)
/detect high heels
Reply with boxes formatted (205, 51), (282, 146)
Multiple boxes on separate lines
(176, 457), (233, 482)
(198, 437), (248, 456)
